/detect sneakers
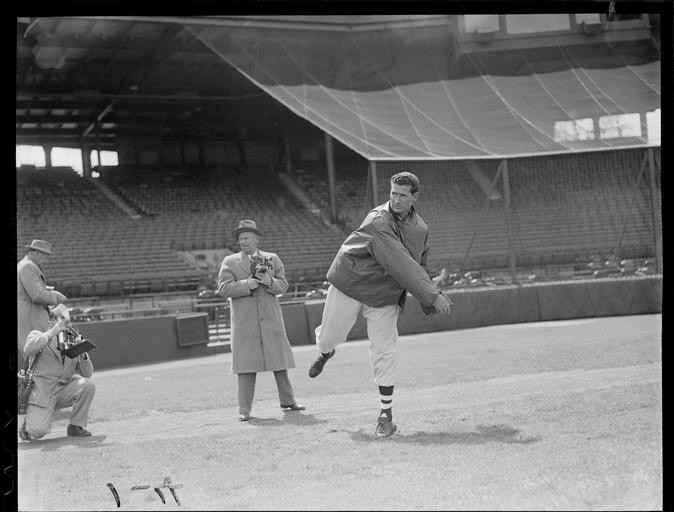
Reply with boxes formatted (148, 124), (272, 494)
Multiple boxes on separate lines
(67, 424), (91, 436)
(19, 416), (31, 440)
(374, 411), (396, 438)
(309, 348), (335, 378)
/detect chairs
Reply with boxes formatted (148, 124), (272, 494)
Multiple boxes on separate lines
(80, 282), (124, 297)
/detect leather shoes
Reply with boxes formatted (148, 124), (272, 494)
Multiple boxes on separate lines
(280, 401), (306, 410)
(238, 413), (250, 420)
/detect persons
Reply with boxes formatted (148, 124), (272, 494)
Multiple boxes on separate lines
(308, 172), (454, 441)
(16, 238), (69, 373)
(218, 220), (306, 421)
(19, 301), (96, 440)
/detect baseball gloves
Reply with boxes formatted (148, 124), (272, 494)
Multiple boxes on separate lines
(420, 288), (453, 315)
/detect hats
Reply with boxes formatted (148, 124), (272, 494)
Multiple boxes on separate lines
(26, 239), (52, 255)
(232, 219), (264, 240)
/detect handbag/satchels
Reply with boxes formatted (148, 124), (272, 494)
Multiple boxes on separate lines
(18, 369), (35, 415)
